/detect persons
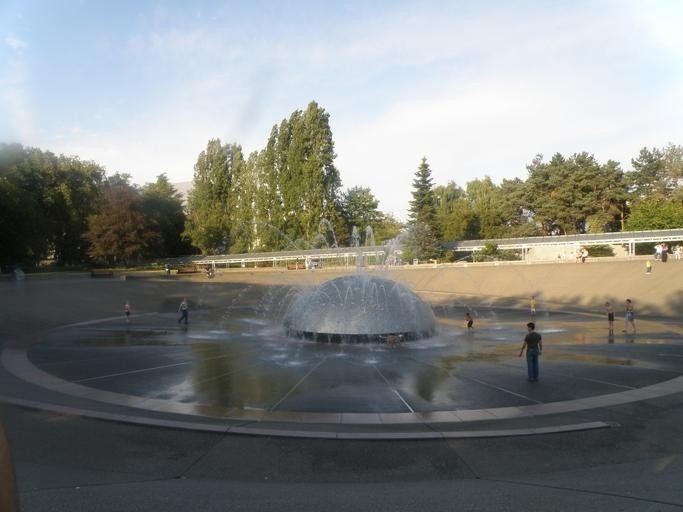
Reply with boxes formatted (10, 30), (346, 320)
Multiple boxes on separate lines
(607, 329), (614, 344)
(604, 302), (614, 329)
(644, 259), (651, 272)
(581, 248), (588, 263)
(620, 299), (635, 334)
(623, 332), (637, 345)
(652, 242), (680, 263)
(517, 322), (542, 383)
(123, 299), (130, 323)
(529, 296), (535, 321)
(464, 313), (473, 329)
(176, 296), (188, 325)
